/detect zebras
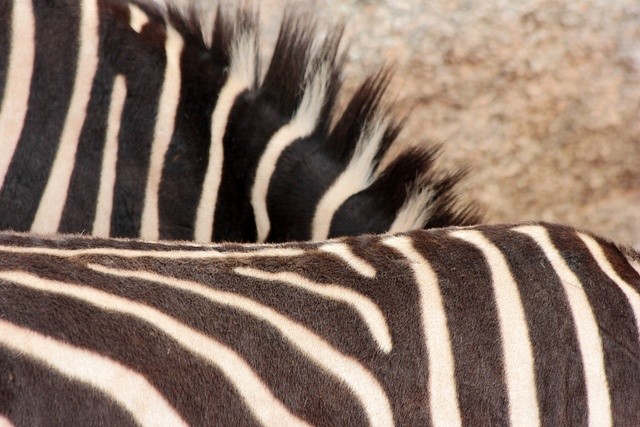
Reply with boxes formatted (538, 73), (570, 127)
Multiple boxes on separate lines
(1, 0), (489, 242)
(0, 222), (640, 427)
